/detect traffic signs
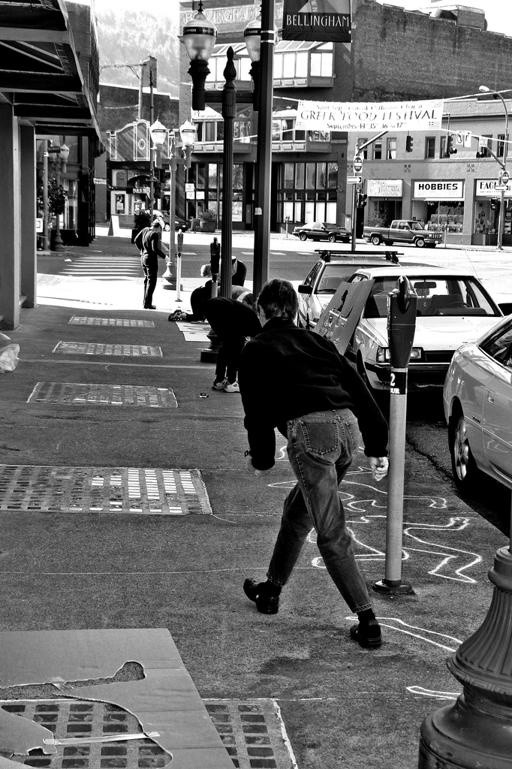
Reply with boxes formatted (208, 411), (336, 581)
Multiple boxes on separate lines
(347, 176), (363, 184)
(494, 184), (508, 191)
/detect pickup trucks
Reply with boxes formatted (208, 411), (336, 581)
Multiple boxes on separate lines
(363, 216), (444, 249)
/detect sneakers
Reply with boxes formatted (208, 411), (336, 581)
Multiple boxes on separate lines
(210, 376), (241, 396)
(143, 302), (185, 322)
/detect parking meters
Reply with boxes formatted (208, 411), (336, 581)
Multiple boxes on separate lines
(373, 273), (419, 598)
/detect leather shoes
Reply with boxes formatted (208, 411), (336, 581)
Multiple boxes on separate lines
(346, 620), (390, 649)
(242, 576), (280, 614)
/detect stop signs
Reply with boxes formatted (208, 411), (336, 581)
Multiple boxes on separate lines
(353, 155), (364, 173)
(501, 170), (509, 184)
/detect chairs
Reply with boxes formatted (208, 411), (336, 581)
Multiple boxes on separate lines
(374, 295), (387, 317)
(430, 294), (462, 309)
(416, 288), (430, 311)
(436, 308), (487, 316)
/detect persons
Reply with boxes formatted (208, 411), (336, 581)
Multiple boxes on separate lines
(238, 279), (389, 649)
(168, 255), (260, 394)
(133, 219), (170, 309)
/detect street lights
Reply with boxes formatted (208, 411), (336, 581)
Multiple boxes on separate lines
(47, 141), (70, 252)
(146, 114), (198, 291)
(479, 84), (509, 251)
(179, 1), (261, 362)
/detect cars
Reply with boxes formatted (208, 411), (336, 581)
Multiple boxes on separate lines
(144, 209), (189, 232)
(340, 264), (509, 406)
(292, 221), (351, 245)
(296, 248), (403, 335)
(443, 311), (512, 501)
